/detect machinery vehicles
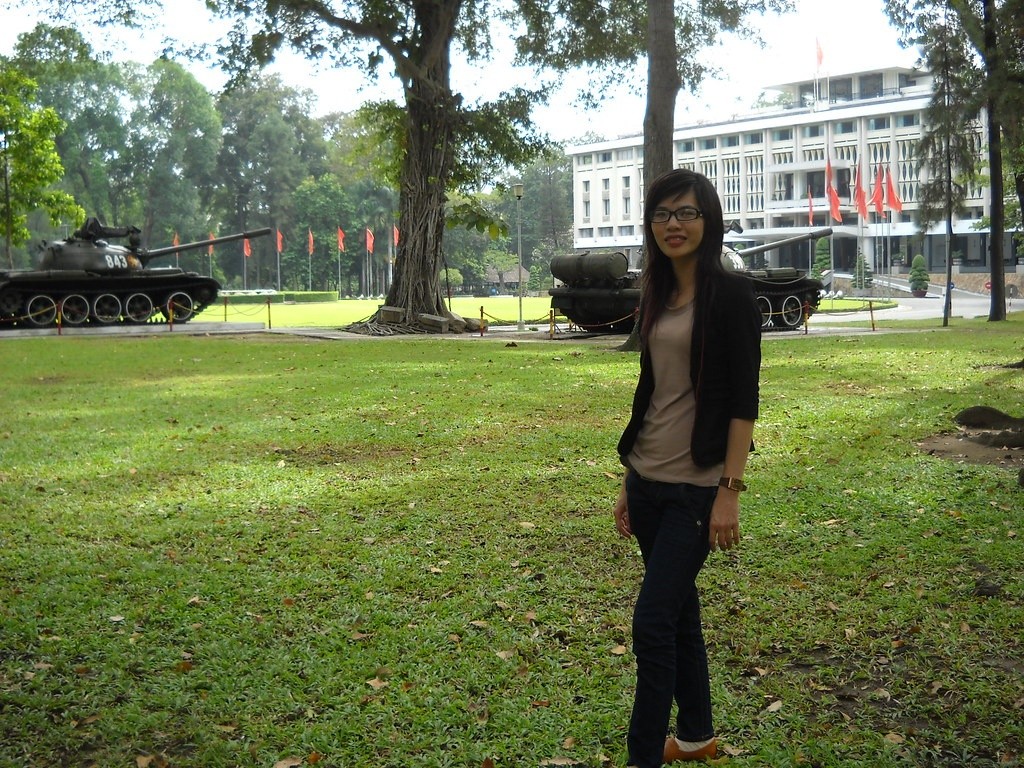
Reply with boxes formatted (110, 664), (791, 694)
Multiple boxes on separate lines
(0, 217), (273, 330)
(548, 220), (834, 334)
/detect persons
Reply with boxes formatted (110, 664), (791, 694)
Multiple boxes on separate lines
(613, 170), (763, 768)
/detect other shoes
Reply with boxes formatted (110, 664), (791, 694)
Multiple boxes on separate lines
(663, 737), (718, 764)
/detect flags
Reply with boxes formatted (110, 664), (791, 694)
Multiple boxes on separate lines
(174, 234), (179, 254)
(367, 230), (375, 253)
(277, 230), (283, 253)
(338, 228), (345, 253)
(209, 234), (214, 254)
(809, 194), (813, 226)
(309, 233), (314, 255)
(826, 162), (842, 222)
(244, 238), (252, 256)
(856, 160), (902, 220)
(393, 227), (400, 246)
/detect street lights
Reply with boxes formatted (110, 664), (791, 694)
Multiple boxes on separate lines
(513, 184), (525, 330)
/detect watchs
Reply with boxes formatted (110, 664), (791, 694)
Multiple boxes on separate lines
(717, 477), (748, 492)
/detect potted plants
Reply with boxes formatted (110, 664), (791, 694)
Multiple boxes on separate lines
(850, 246), (874, 297)
(892, 251), (905, 266)
(908, 254), (930, 298)
(1015, 244), (1024, 265)
(952, 249), (964, 265)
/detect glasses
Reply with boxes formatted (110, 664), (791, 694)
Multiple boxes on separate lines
(647, 206), (703, 224)
